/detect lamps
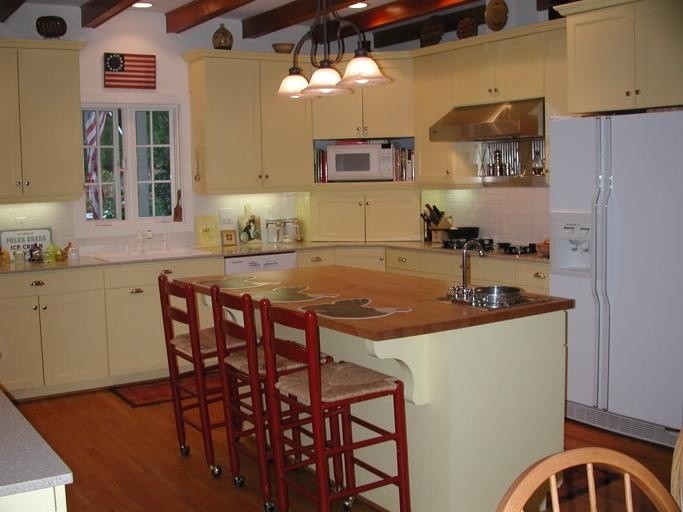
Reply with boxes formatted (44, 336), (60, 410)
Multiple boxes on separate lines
(265, 0), (392, 102)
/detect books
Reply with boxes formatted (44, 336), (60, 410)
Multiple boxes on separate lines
(392, 143), (415, 182)
(313, 139), (328, 184)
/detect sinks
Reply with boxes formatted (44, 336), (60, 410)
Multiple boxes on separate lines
(431, 286), (551, 310)
(89, 248), (214, 262)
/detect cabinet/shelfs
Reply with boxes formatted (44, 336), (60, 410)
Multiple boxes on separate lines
(553, 0), (683, 117)
(465, 256), (553, 298)
(312, 178), (428, 246)
(0, 483), (69, 512)
(378, 244), (469, 285)
(305, 46), (414, 141)
(0, 237), (228, 404)
(0, 39), (90, 203)
(409, 40), (481, 193)
(295, 245), (386, 268)
(542, 20), (568, 187)
(450, 26), (543, 108)
(186, 42), (316, 197)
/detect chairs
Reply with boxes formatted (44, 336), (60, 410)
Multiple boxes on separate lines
(494, 445), (679, 512)
(155, 271), (282, 477)
(208, 283), (350, 512)
(257, 292), (414, 511)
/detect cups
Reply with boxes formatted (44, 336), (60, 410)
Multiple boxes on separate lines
(506, 244), (535, 254)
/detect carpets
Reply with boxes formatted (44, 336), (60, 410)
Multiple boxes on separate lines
(110, 365), (233, 407)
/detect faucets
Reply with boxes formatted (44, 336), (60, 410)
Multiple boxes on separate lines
(136, 229), (152, 253)
(461, 240), (485, 299)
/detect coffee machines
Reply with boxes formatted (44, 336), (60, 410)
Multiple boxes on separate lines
(265, 218), (299, 241)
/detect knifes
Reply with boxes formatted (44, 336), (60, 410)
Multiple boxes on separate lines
(419, 203), (443, 226)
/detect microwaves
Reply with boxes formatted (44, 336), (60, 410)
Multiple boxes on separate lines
(326, 143), (396, 181)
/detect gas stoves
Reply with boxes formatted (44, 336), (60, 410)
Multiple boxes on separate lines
(441, 239), (493, 248)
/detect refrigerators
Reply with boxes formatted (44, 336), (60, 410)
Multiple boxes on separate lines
(548, 110), (682, 449)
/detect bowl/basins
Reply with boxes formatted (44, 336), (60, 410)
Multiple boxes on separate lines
(497, 243), (510, 250)
(536, 242), (549, 257)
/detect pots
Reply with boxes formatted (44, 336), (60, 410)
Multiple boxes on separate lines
(426, 227), (478, 239)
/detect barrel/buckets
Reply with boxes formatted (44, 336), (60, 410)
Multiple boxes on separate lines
(476, 286), (521, 310)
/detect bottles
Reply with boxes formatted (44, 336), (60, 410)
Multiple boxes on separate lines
(12, 243), (80, 267)
(489, 149), (545, 176)
